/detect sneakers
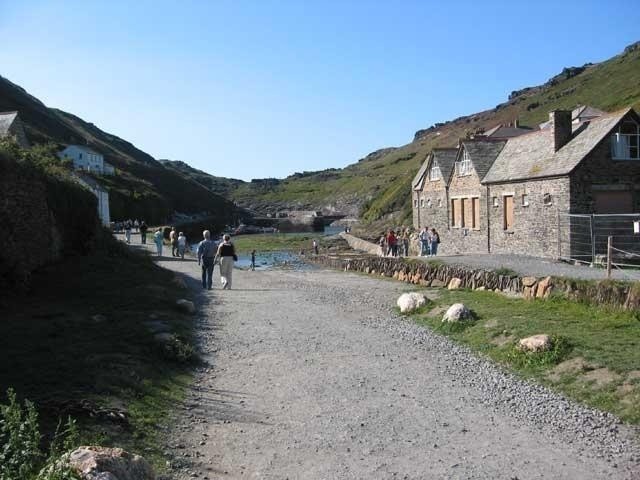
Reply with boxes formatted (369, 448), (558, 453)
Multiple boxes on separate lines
(200, 286), (211, 290)
(223, 281), (232, 290)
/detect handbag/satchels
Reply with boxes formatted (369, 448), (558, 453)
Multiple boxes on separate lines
(234, 254), (239, 261)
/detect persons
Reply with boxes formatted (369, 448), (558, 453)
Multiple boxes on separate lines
(251, 250), (256, 271)
(114, 218), (187, 260)
(379, 226), (441, 258)
(313, 240), (319, 254)
(196, 230), (218, 290)
(213, 233), (238, 291)
